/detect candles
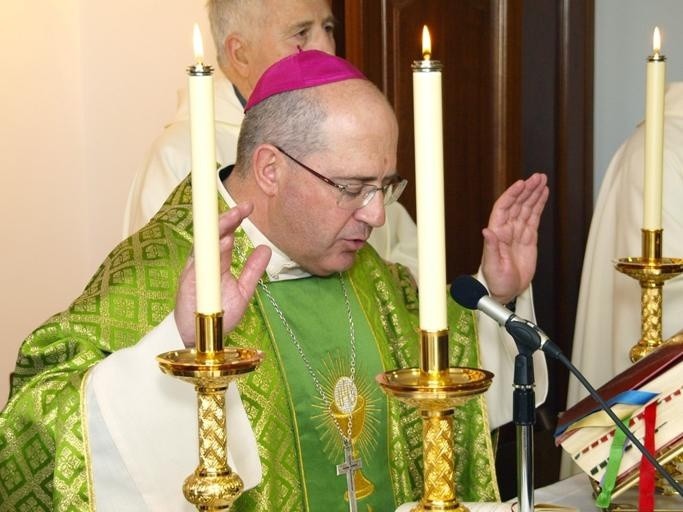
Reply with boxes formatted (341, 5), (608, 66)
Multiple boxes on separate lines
(184, 21), (222, 317)
(408, 22), (450, 332)
(637, 24), (666, 231)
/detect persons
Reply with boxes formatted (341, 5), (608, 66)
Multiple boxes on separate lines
(0, 52), (549, 512)
(121, 0), (419, 288)
(556, 83), (683, 480)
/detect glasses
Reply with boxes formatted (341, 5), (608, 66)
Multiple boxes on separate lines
(272, 142), (408, 211)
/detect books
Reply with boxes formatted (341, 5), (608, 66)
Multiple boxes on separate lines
(549, 330), (683, 496)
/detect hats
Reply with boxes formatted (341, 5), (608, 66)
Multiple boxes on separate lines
(243, 44), (368, 115)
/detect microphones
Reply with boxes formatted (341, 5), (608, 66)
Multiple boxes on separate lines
(450, 274), (562, 360)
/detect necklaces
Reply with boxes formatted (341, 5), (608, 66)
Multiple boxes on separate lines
(230, 233), (363, 512)
